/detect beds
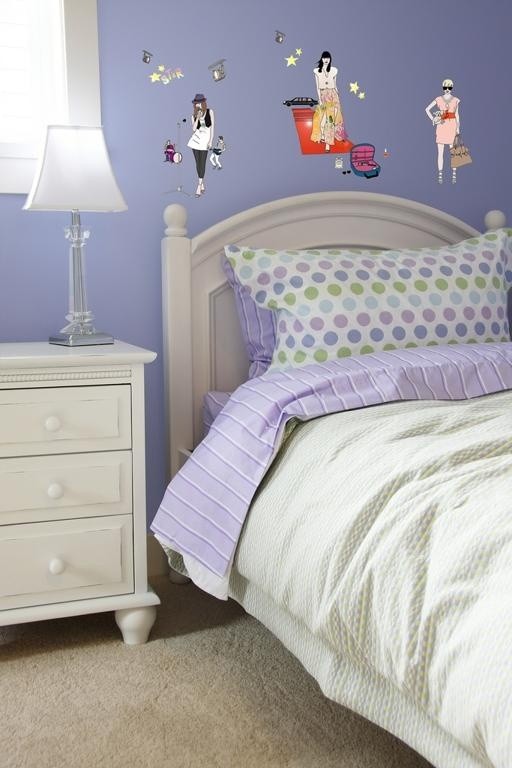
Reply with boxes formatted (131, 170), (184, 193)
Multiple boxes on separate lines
(161, 191), (511, 768)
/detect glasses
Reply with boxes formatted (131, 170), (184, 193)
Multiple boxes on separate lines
(343, 171), (351, 174)
(443, 87), (453, 90)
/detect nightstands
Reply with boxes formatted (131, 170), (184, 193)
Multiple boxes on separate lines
(0, 340), (160, 648)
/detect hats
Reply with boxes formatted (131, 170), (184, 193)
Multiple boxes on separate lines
(192, 94), (206, 103)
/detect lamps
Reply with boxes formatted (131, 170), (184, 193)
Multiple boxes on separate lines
(21, 126), (130, 346)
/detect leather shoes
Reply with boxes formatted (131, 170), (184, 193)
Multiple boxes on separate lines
(195, 190), (205, 198)
(321, 139), (330, 152)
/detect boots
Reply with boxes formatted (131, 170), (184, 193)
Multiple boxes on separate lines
(452, 170), (456, 184)
(438, 170), (443, 184)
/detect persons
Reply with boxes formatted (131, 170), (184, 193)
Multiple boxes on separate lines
(424, 78), (461, 185)
(186, 94), (214, 197)
(309, 51), (349, 154)
(209, 135), (225, 171)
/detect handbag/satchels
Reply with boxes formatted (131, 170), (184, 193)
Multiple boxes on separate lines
(450, 146), (472, 168)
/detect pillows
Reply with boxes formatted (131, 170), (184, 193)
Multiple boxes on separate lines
(223, 229), (511, 380)
